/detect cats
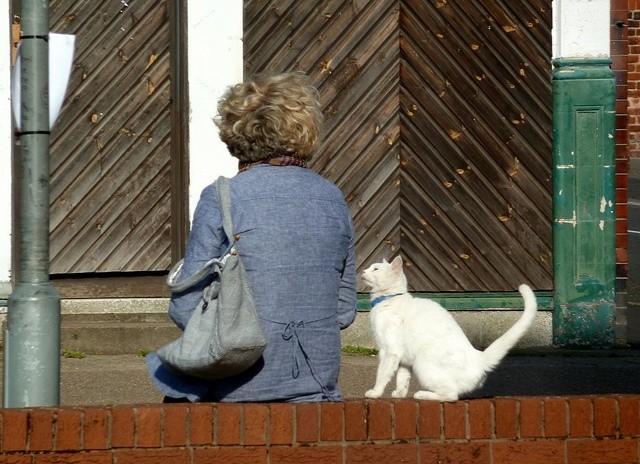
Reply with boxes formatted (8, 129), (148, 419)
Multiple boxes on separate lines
(359, 255), (538, 401)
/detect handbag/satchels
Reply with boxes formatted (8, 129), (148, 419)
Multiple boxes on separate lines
(156, 176), (267, 381)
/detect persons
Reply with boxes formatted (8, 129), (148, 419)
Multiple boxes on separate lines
(149, 74), (357, 402)
